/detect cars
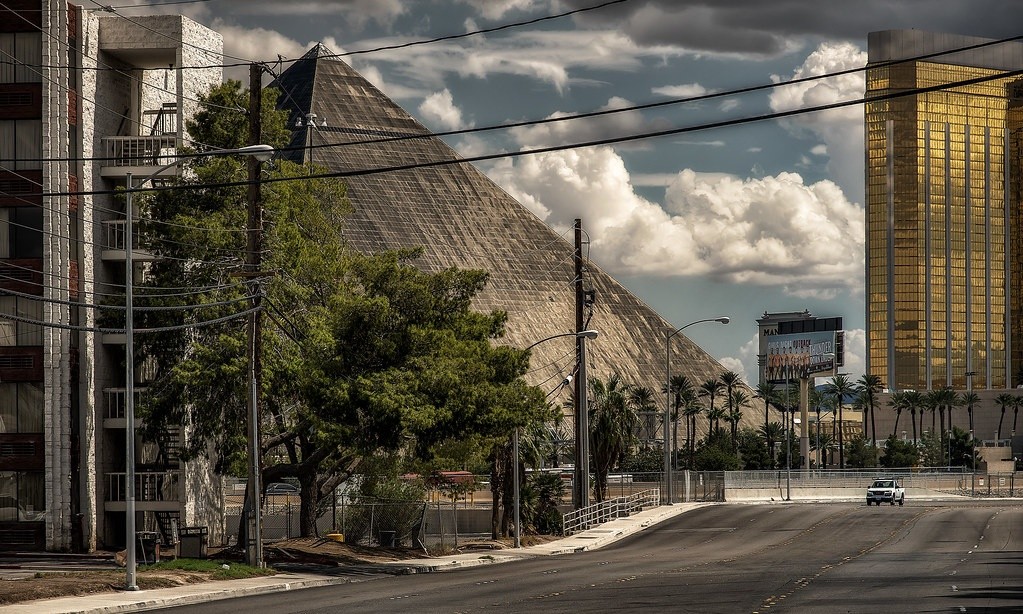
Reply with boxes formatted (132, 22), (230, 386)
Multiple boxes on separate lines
(265, 483), (302, 496)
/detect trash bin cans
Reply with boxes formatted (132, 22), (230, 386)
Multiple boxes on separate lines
(378, 530), (396, 547)
(135, 531), (161, 564)
(411, 522), (428, 550)
(326, 534), (344, 542)
(715, 476), (726, 502)
(179, 526), (208, 558)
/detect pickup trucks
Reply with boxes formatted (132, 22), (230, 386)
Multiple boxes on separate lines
(866, 479), (906, 506)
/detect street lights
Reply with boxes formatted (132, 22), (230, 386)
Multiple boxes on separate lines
(124, 142), (277, 593)
(666, 317), (731, 506)
(786, 352), (837, 502)
(510, 329), (599, 547)
